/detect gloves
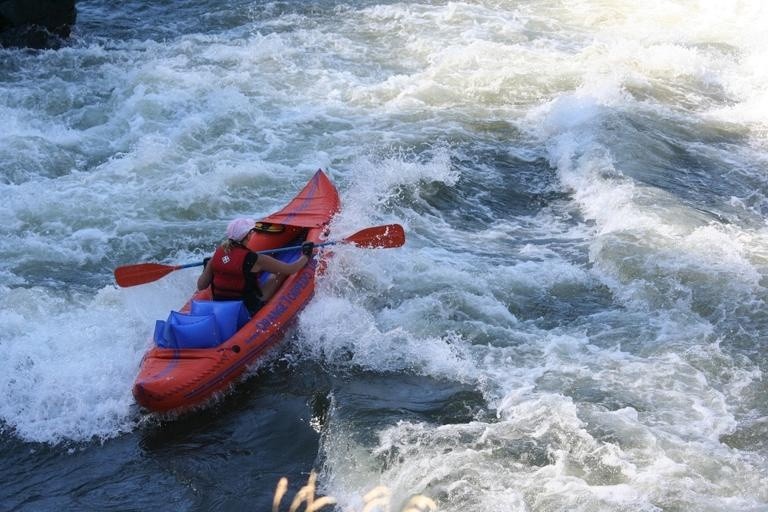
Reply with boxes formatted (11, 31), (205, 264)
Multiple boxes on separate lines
(203, 257), (211, 272)
(302, 241), (313, 255)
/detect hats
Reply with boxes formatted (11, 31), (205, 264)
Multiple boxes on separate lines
(226, 218), (255, 242)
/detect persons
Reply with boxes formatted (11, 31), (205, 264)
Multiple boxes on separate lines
(197, 219), (314, 309)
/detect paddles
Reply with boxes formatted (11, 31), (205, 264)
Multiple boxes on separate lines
(114, 224), (405, 287)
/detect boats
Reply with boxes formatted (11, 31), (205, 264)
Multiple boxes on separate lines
(132, 167), (343, 412)
